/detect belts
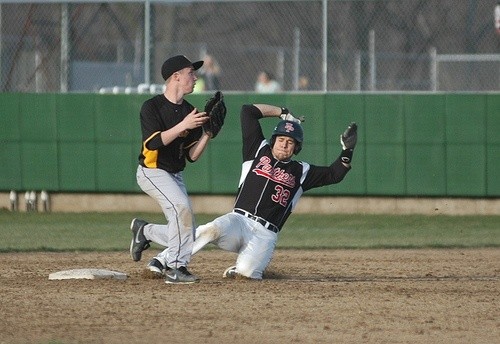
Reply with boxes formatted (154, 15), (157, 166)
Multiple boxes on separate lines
(235, 210), (278, 234)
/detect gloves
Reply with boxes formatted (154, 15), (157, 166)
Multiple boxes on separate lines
(280, 107), (305, 125)
(340, 122), (358, 164)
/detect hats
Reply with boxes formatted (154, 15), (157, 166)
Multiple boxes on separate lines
(161, 55), (205, 81)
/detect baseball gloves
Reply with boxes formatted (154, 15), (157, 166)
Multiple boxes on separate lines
(203, 91), (227, 139)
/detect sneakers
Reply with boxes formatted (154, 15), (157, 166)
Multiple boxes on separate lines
(145, 259), (164, 277)
(130, 218), (152, 262)
(164, 265), (201, 284)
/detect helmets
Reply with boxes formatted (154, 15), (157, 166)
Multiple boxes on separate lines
(270, 120), (304, 156)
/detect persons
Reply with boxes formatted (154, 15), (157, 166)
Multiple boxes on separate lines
(146, 103), (358, 280)
(191, 54), (311, 93)
(130, 55), (213, 285)
